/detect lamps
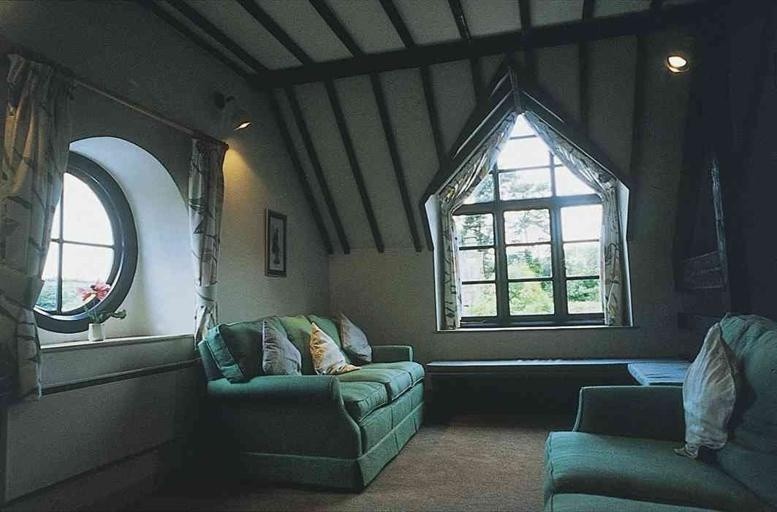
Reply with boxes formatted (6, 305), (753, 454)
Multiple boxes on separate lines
(216, 90), (251, 131)
(664, 22), (695, 75)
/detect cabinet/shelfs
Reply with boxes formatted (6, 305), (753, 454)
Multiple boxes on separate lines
(426, 357), (685, 425)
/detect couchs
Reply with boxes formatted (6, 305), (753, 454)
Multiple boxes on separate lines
(544, 313), (777, 512)
(198, 314), (425, 492)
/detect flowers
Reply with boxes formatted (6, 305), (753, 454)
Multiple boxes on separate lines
(77, 279), (127, 325)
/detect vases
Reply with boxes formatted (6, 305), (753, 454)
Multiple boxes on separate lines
(88, 322), (105, 341)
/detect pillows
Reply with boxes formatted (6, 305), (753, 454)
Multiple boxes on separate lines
(674, 321), (740, 461)
(339, 312), (372, 364)
(262, 320), (302, 376)
(310, 321), (361, 375)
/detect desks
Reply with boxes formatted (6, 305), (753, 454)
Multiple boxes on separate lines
(626, 362), (694, 385)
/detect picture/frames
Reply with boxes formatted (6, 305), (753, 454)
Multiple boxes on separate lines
(265, 208), (287, 277)
